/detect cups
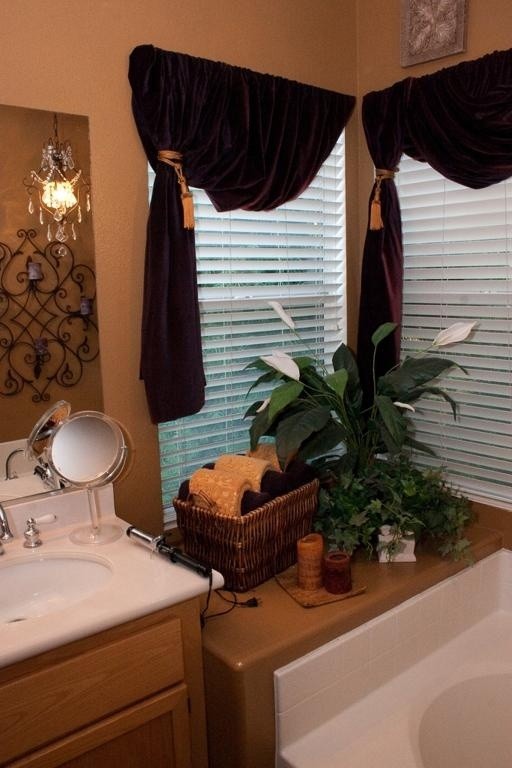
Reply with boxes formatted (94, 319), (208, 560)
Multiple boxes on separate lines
(297, 533), (352, 594)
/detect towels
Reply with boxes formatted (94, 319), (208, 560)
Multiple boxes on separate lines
(246, 441), (308, 471)
(189, 469), (254, 516)
(214, 453), (274, 491)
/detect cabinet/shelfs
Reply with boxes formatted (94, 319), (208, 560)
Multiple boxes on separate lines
(0, 595), (209, 768)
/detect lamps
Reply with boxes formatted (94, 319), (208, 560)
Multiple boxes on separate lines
(26, 261), (43, 290)
(22, 112), (91, 245)
(32, 335), (50, 378)
(79, 298), (92, 331)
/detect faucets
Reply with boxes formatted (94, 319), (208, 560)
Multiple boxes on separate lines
(0, 504), (13, 544)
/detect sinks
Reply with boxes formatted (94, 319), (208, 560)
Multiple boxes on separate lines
(0, 494), (22, 502)
(409, 659), (512, 768)
(0, 550), (116, 624)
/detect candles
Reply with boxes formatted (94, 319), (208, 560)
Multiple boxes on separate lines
(297, 533), (324, 591)
(324, 552), (351, 595)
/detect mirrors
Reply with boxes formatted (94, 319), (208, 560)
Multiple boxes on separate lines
(47, 408), (136, 546)
(23, 398), (71, 478)
(0, 102), (106, 510)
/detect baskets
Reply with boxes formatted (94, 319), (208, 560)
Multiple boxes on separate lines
(173, 454), (318, 595)
